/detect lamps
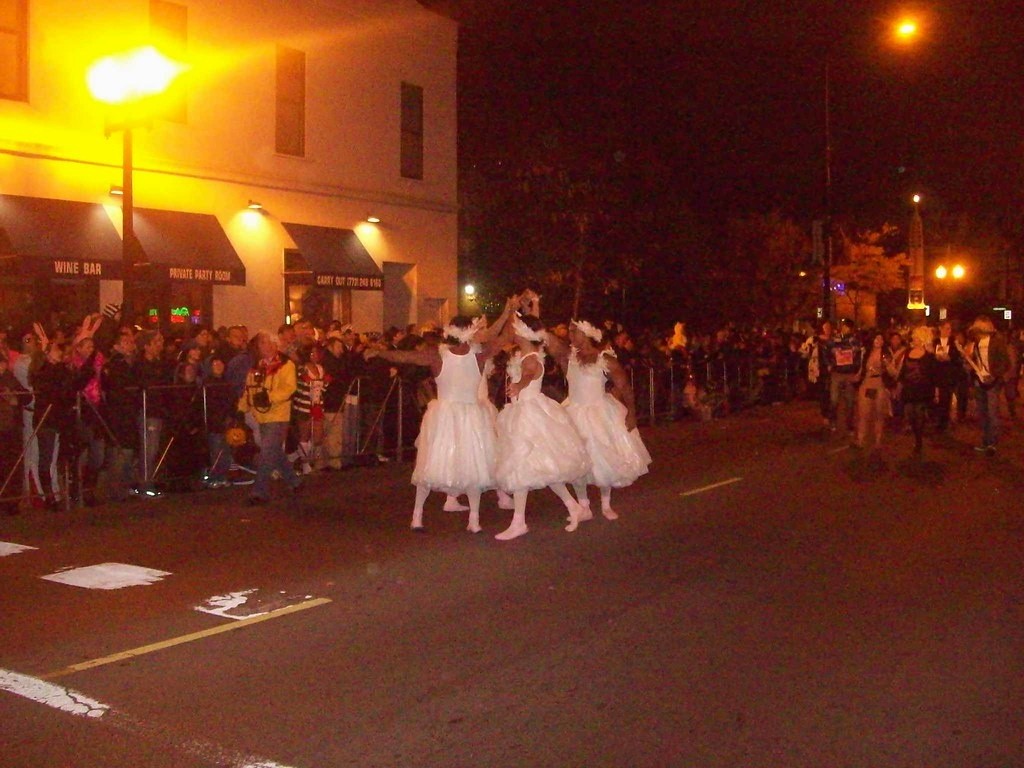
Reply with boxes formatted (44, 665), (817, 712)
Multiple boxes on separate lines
(248, 199), (262, 208)
(110, 186), (123, 195)
(367, 215), (381, 223)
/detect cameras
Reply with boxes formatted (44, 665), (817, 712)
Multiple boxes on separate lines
(252, 370), (266, 382)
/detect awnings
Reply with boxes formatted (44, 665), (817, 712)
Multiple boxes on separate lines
(121, 206), (247, 286)
(0, 194), (131, 281)
(280, 221), (385, 290)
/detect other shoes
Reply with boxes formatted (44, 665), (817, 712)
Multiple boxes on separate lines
(567, 509), (593, 521)
(410, 521), (423, 529)
(495, 524), (529, 540)
(466, 525), (481, 533)
(848, 442), (863, 450)
(291, 478), (304, 490)
(564, 505), (583, 531)
(444, 503), (469, 511)
(498, 499), (515, 509)
(602, 509), (619, 520)
(973, 444), (996, 457)
(240, 494), (267, 503)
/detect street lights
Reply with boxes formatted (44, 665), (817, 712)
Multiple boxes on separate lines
(822, 19), (918, 318)
(90, 53), (174, 326)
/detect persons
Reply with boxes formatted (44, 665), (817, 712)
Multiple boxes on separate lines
(0, 288), (1024, 539)
